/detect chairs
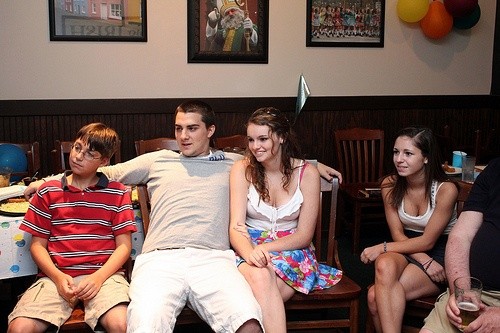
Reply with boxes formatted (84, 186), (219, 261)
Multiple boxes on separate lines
(137, 182), (206, 326)
(334, 127), (385, 261)
(285, 176), (361, 333)
(213, 134), (248, 152)
(368, 182), (474, 329)
(55, 139), (120, 172)
(0, 140), (41, 181)
(133, 138), (181, 155)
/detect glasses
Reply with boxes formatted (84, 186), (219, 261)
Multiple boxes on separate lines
(70, 144), (99, 161)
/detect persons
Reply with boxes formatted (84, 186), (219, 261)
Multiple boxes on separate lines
(358, 125), (459, 333)
(419, 158), (500, 333)
(312, 3), (380, 39)
(207, 0), (258, 54)
(24, 102), (343, 333)
(7, 122), (138, 333)
(228, 108), (342, 333)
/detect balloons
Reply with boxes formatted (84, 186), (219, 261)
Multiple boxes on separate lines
(396, 0), (481, 39)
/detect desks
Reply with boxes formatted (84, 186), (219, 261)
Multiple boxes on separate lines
(0, 187), (145, 279)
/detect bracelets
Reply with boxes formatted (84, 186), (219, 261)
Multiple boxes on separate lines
(384, 241), (387, 253)
(422, 257), (434, 270)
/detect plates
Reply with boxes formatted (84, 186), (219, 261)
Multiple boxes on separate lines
(442, 165), (463, 174)
(0, 194), (33, 217)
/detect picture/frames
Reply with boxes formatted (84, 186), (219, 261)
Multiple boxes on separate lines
(186, 0), (269, 65)
(306, 0), (385, 49)
(48, 0), (148, 42)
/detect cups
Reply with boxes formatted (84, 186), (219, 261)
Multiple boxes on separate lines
(462, 156), (476, 181)
(24, 175), (37, 186)
(453, 277), (483, 331)
(0, 167), (14, 188)
(452, 151), (468, 168)
